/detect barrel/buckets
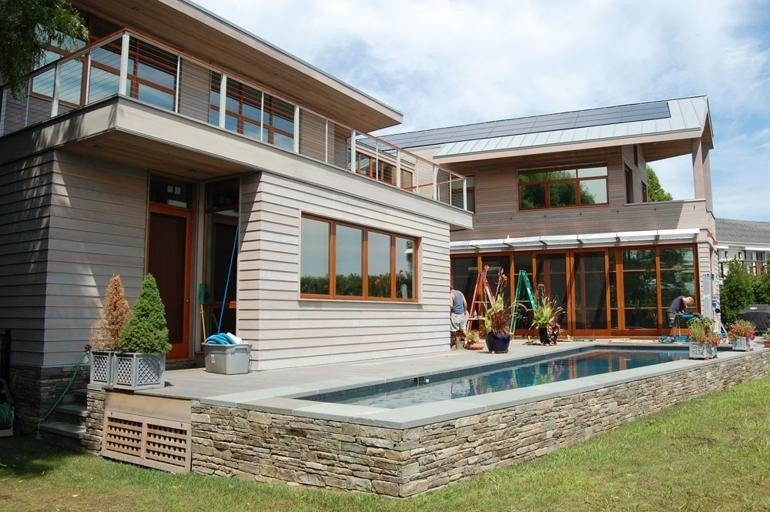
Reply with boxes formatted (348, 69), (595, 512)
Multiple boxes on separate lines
(1, 401), (15, 428)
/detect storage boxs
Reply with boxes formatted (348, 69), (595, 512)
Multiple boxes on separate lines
(200, 342), (252, 375)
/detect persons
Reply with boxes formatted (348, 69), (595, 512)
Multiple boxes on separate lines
(450, 284), (468, 344)
(667, 295), (695, 342)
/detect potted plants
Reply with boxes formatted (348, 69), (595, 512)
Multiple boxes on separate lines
(685, 317), (721, 359)
(527, 296), (564, 346)
(485, 293), (518, 353)
(466, 331), (484, 350)
(88, 272), (174, 393)
(764, 328), (770, 348)
(727, 318), (758, 351)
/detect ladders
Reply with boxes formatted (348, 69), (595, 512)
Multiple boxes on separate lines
(509, 270), (539, 337)
(466, 269), (496, 332)
